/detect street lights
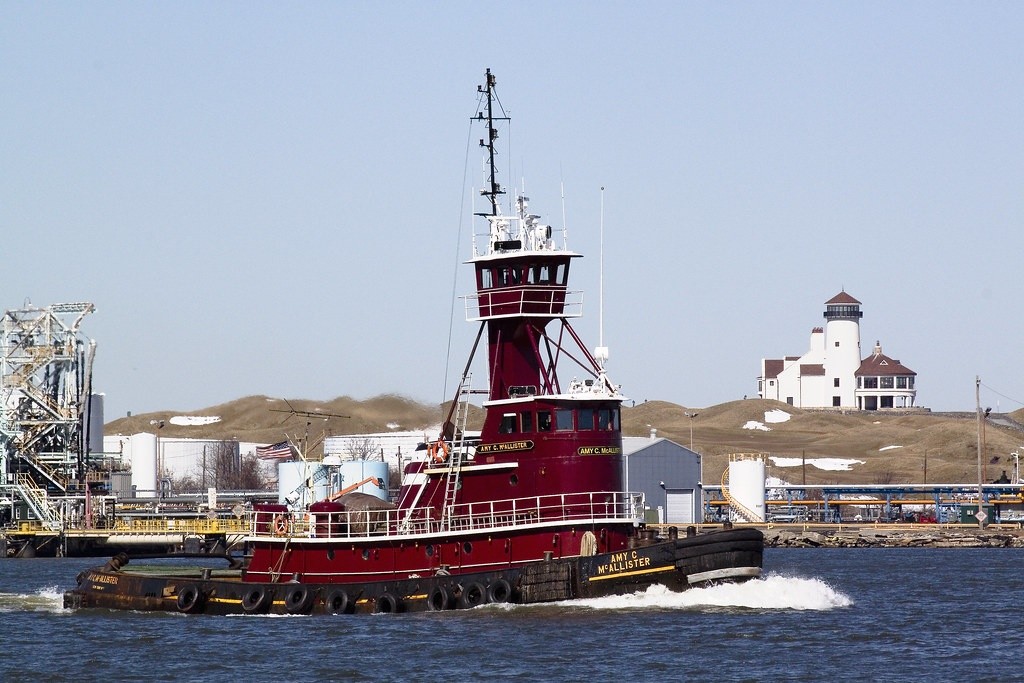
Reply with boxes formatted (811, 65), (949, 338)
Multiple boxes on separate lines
(681, 411), (701, 450)
(981, 407), (993, 485)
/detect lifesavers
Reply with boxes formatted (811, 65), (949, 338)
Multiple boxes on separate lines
(375, 594), (398, 615)
(241, 585), (274, 614)
(324, 589), (356, 615)
(460, 582), (487, 610)
(433, 442), (448, 462)
(275, 514), (288, 536)
(176, 585), (204, 613)
(285, 584), (313, 614)
(428, 587), (449, 612)
(488, 579), (513, 604)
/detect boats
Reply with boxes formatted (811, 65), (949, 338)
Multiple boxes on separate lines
(61, 62), (777, 612)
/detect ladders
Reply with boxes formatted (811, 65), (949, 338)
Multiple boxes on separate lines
(438, 369), (475, 532)
